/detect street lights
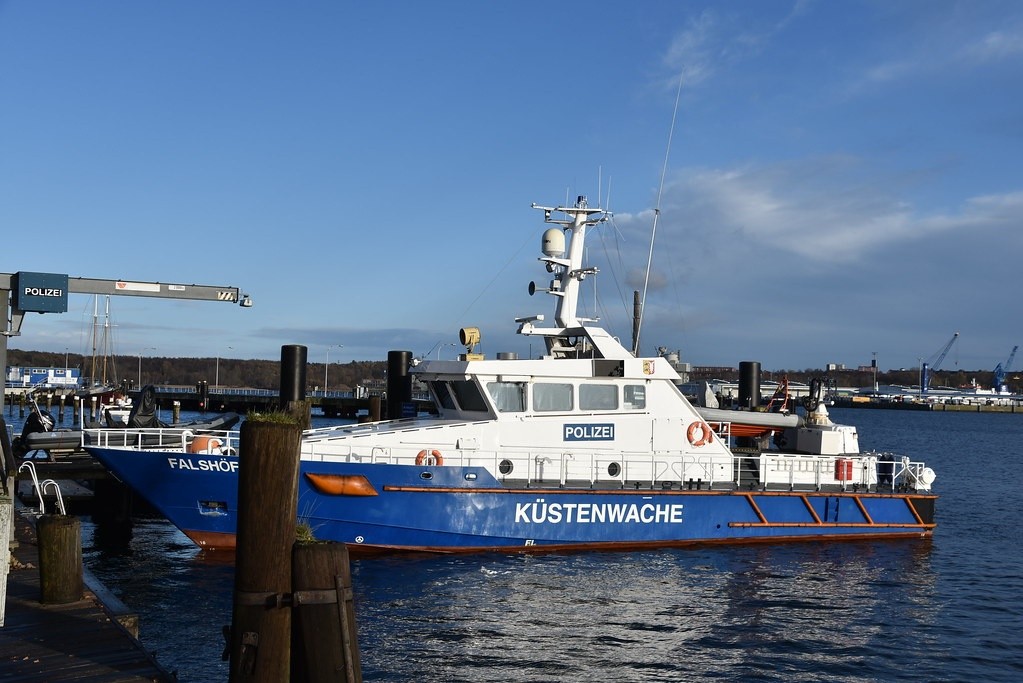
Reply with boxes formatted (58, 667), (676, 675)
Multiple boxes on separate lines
(217, 346), (235, 387)
(138, 347), (157, 391)
(324, 346), (343, 397)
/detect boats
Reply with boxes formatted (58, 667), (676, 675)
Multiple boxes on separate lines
(81, 64), (934, 551)
(0, 384), (238, 499)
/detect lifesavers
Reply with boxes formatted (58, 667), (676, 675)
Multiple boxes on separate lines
(414, 448), (444, 467)
(687, 420), (710, 447)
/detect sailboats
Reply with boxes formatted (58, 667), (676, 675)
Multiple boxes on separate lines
(77, 294), (135, 409)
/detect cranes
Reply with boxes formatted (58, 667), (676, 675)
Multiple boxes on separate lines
(993, 346), (1018, 392)
(922, 332), (959, 394)
